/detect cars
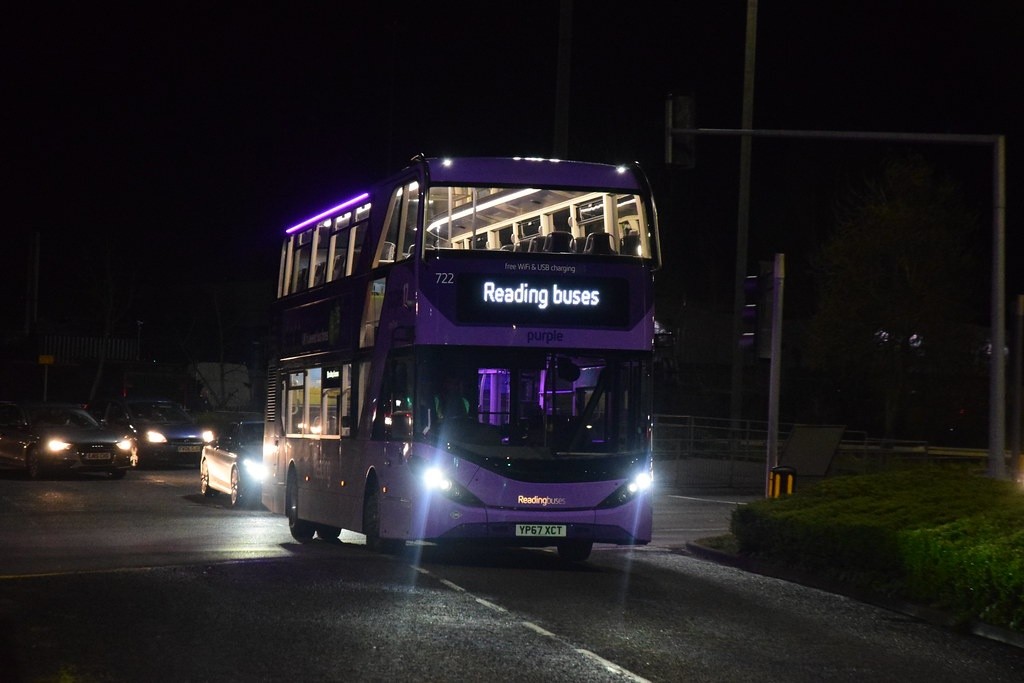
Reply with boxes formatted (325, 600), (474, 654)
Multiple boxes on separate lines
(0, 400), (138, 480)
(89, 397), (204, 470)
(200, 420), (269, 508)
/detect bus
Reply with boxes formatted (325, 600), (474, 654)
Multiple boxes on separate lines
(258, 151), (677, 564)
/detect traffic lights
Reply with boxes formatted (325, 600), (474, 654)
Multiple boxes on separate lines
(738, 270), (772, 370)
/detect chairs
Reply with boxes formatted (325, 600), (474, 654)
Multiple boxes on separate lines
(313, 414), (338, 435)
(294, 241), (434, 292)
(498, 231), (642, 255)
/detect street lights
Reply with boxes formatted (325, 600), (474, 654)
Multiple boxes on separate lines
(39, 355), (55, 403)
